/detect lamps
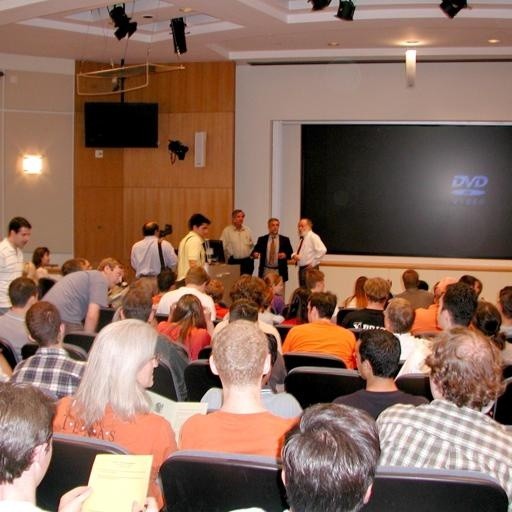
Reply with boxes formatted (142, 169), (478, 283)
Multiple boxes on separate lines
(169, 17), (190, 54)
(439, 0), (467, 20)
(334, 0), (356, 21)
(106, 3), (137, 40)
(405, 49), (417, 87)
(167, 138), (189, 164)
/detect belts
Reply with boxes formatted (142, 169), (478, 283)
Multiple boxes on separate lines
(265, 265), (278, 269)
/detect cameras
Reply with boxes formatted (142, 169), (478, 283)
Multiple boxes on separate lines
(160, 224), (173, 236)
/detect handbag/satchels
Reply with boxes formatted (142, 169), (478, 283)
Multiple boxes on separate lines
(157, 239), (175, 292)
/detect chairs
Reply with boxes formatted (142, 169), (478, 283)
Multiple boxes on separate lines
(0, 277), (512, 512)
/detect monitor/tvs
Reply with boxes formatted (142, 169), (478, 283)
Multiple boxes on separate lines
(84, 101), (159, 148)
(205, 239), (225, 264)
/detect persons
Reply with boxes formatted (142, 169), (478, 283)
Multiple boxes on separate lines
(374, 328), (512, 510)
(2, 216), (512, 424)
(177, 319), (291, 461)
(51, 318), (178, 509)
(279, 403), (382, 511)
(130, 211), (328, 305)
(1, 382), (159, 510)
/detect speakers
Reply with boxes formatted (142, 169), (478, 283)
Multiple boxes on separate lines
(194, 132), (207, 167)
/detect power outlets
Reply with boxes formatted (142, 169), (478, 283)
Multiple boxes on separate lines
(95, 150), (103, 158)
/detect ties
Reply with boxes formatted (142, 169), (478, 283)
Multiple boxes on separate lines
(294, 236), (304, 267)
(269, 236), (276, 266)
